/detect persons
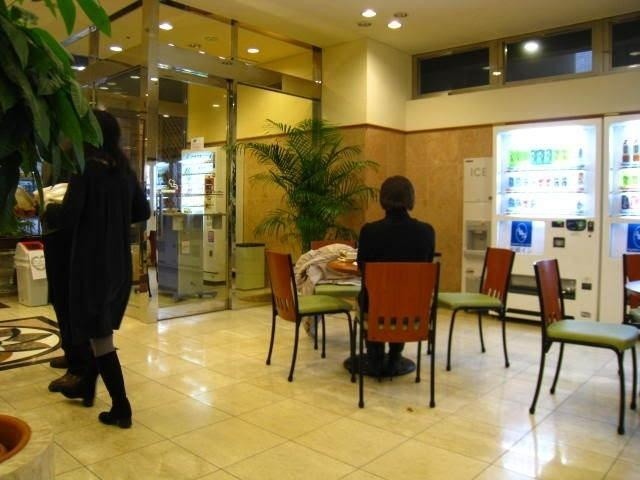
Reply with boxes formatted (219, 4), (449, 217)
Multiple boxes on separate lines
(36, 106), (153, 430)
(10, 128), (81, 396)
(355, 175), (437, 375)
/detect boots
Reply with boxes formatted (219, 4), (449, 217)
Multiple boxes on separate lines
(96, 349), (132, 428)
(62, 352), (98, 407)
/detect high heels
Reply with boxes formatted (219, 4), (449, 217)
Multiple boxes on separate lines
(368, 359), (401, 383)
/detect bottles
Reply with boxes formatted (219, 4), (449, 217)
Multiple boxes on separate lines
(633, 140), (640, 163)
(619, 174), (639, 212)
(506, 150), (585, 214)
(623, 140), (630, 164)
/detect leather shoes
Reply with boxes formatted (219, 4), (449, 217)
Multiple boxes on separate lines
(50, 355), (81, 392)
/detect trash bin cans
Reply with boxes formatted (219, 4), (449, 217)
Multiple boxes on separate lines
(235, 243), (265, 290)
(12, 241), (49, 306)
